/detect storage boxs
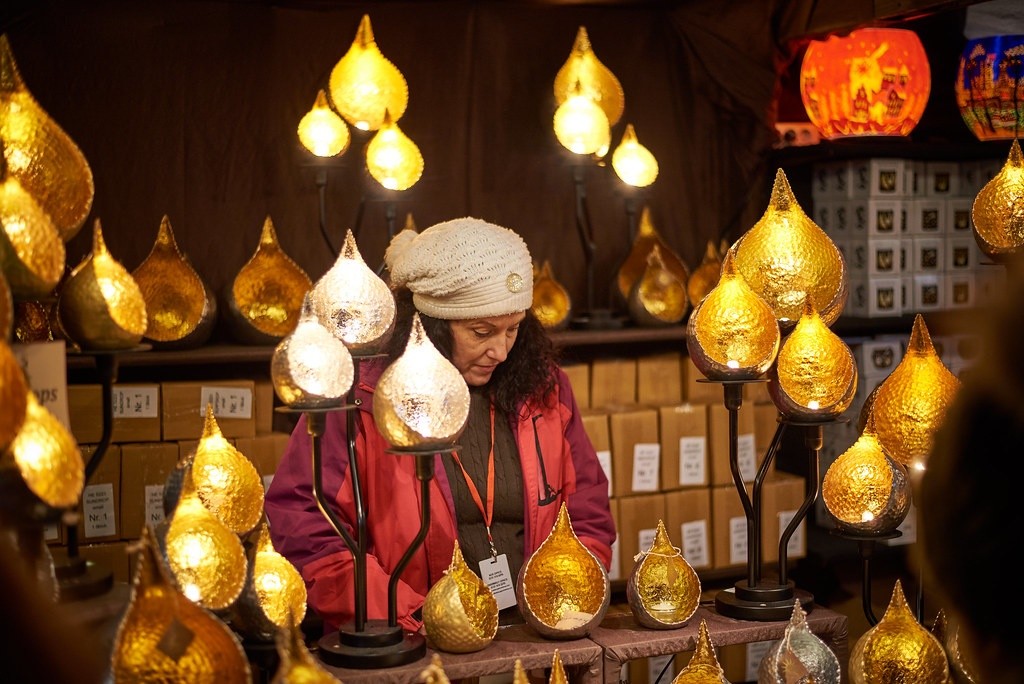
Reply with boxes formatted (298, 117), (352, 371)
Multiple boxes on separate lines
(811, 160), (986, 315)
(44, 353), (807, 580)
(819, 334), (971, 541)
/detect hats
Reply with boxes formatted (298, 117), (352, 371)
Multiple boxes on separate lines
(383, 217), (533, 320)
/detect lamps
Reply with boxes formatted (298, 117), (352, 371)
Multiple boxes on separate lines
(690, 166), (857, 620)
(272, 228), (471, 667)
(550, 24), (658, 334)
(823, 311), (961, 632)
(0, 33), (147, 602)
(298, 13), (423, 278)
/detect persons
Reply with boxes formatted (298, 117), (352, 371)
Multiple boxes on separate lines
(263, 216), (618, 637)
